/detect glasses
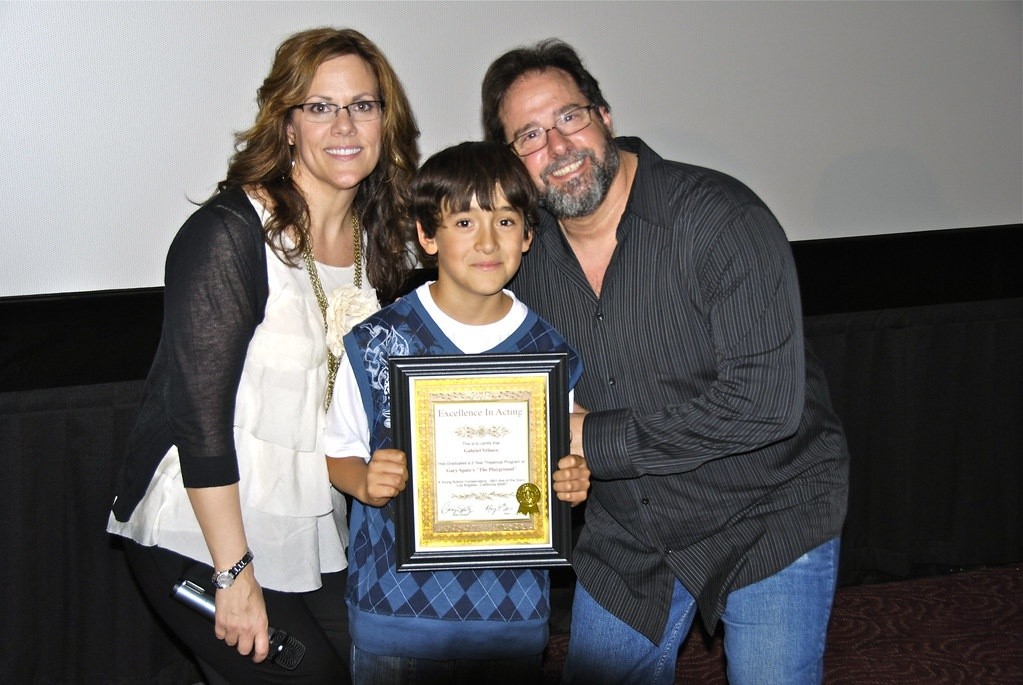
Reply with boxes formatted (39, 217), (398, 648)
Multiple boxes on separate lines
(506, 104), (597, 156)
(287, 100), (385, 122)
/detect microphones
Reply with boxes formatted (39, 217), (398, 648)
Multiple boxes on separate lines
(170, 580), (306, 671)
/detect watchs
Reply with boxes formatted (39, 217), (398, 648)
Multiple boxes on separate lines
(210, 549), (257, 590)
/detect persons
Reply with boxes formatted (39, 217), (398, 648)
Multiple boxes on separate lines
(475, 38), (854, 685)
(98, 25), (432, 684)
(324, 142), (592, 684)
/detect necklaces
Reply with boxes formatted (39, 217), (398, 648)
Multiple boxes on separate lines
(290, 203), (365, 412)
